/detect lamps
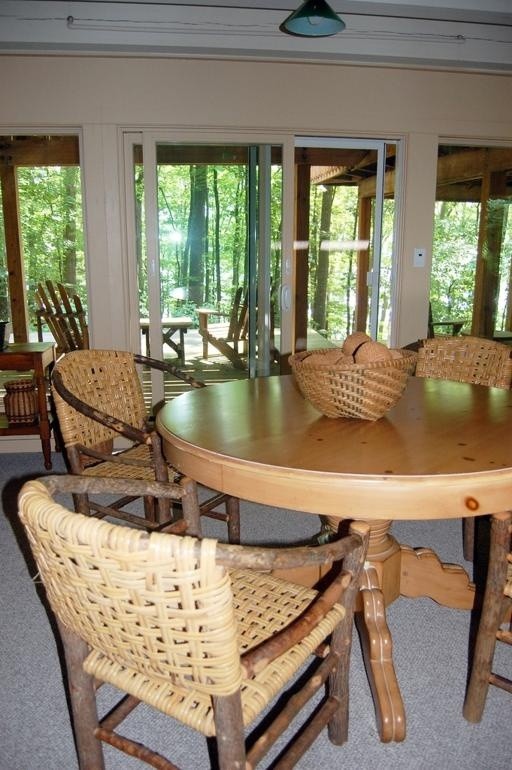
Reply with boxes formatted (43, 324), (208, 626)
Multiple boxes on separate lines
(278, 0), (346, 38)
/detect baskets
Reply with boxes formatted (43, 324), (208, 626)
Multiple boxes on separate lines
(3, 379), (38, 428)
(287, 347), (418, 422)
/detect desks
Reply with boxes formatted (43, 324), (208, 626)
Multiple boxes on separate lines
(139, 315), (194, 362)
(1, 341), (60, 471)
(459, 328), (512, 343)
(155, 368), (512, 740)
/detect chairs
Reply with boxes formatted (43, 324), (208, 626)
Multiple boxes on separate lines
(32, 280), (89, 349)
(461, 500), (510, 721)
(48, 345), (243, 548)
(414, 333), (511, 563)
(197, 274), (275, 370)
(16, 474), (372, 770)
(427, 302), (465, 339)
